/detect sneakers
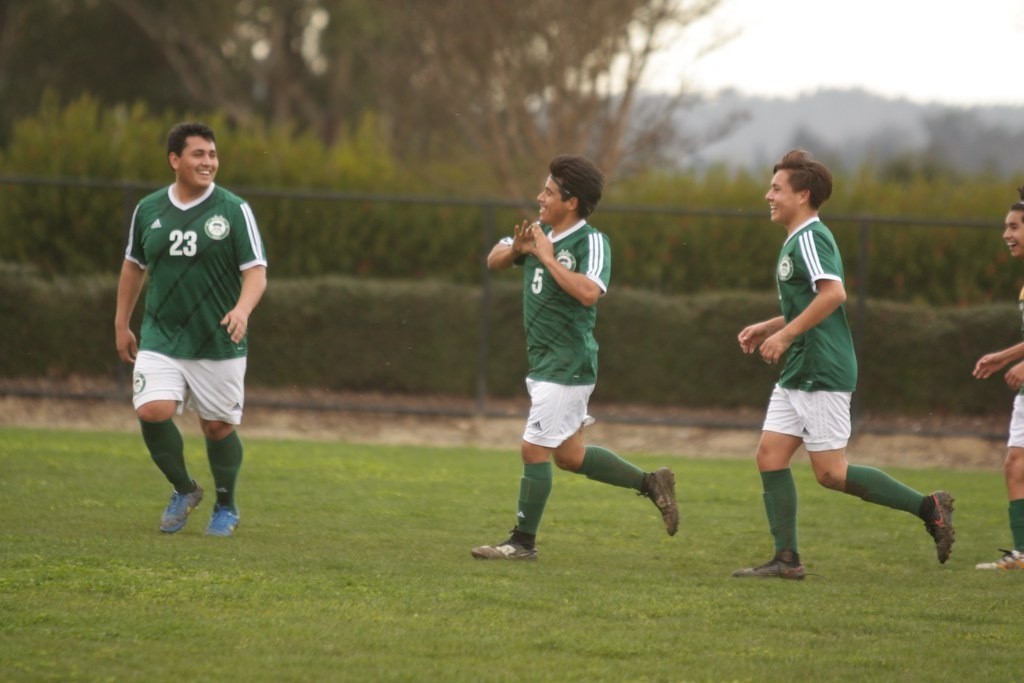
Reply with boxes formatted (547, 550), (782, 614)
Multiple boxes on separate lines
(471, 526), (537, 561)
(730, 550), (806, 580)
(636, 468), (679, 536)
(159, 478), (204, 533)
(207, 503), (240, 536)
(923, 491), (956, 564)
(973, 548), (1024, 570)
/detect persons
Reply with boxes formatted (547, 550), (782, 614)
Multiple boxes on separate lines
(972, 189), (1024, 569)
(732, 150), (956, 578)
(116, 124), (267, 536)
(471, 156), (678, 561)
